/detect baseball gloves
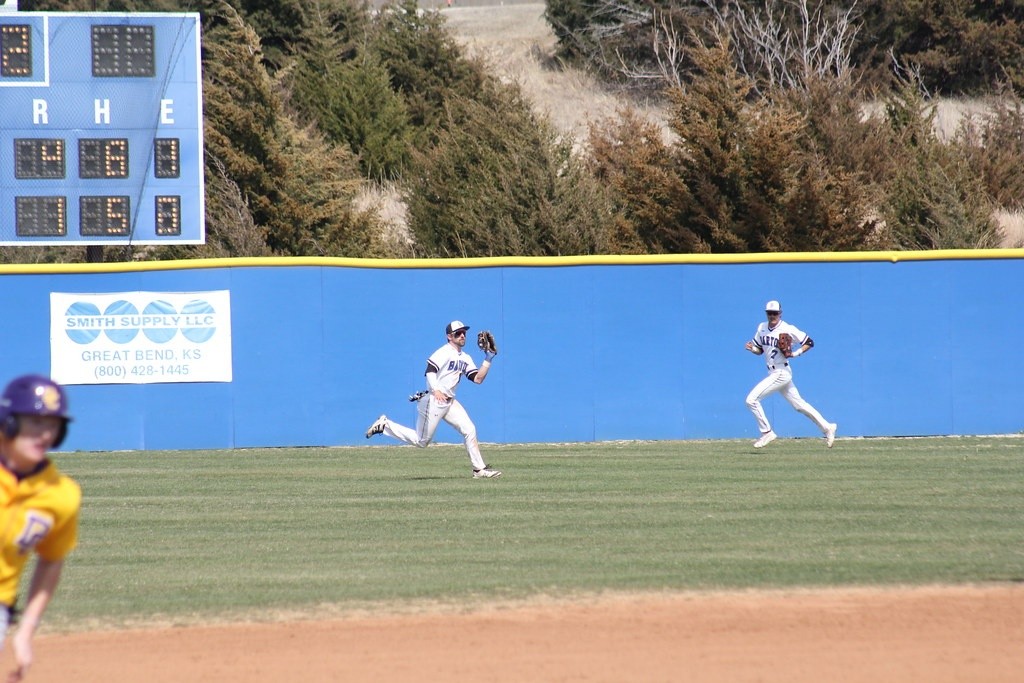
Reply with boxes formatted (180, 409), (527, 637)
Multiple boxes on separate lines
(778, 333), (793, 356)
(477, 331), (497, 355)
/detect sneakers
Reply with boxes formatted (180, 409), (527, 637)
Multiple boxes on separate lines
(753, 431), (777, 447)
(366, 414), (388, 440)
(826, 424), (837, 448)
(473, 464), (501, 479)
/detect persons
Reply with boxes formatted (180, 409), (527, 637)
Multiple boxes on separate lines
(0, 376), (83, 683)
(366, 320), (501, 479)
(745, 300), (837, 448)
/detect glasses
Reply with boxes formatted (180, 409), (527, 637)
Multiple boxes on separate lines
(767, 312), (778, 316)
(451, 330), (466, 338)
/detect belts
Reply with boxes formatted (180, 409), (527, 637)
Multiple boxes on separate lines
(767, 363), (788, 370)
(446, 397), (451, 401)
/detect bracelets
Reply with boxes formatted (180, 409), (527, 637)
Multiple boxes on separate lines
(482, 360), (490, 368)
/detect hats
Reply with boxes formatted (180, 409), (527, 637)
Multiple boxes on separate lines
(765, 301), (781, 311)
(446, 321), (471, 333)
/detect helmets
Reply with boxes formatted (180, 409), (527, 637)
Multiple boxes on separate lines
(0, 374), (75, 449)
